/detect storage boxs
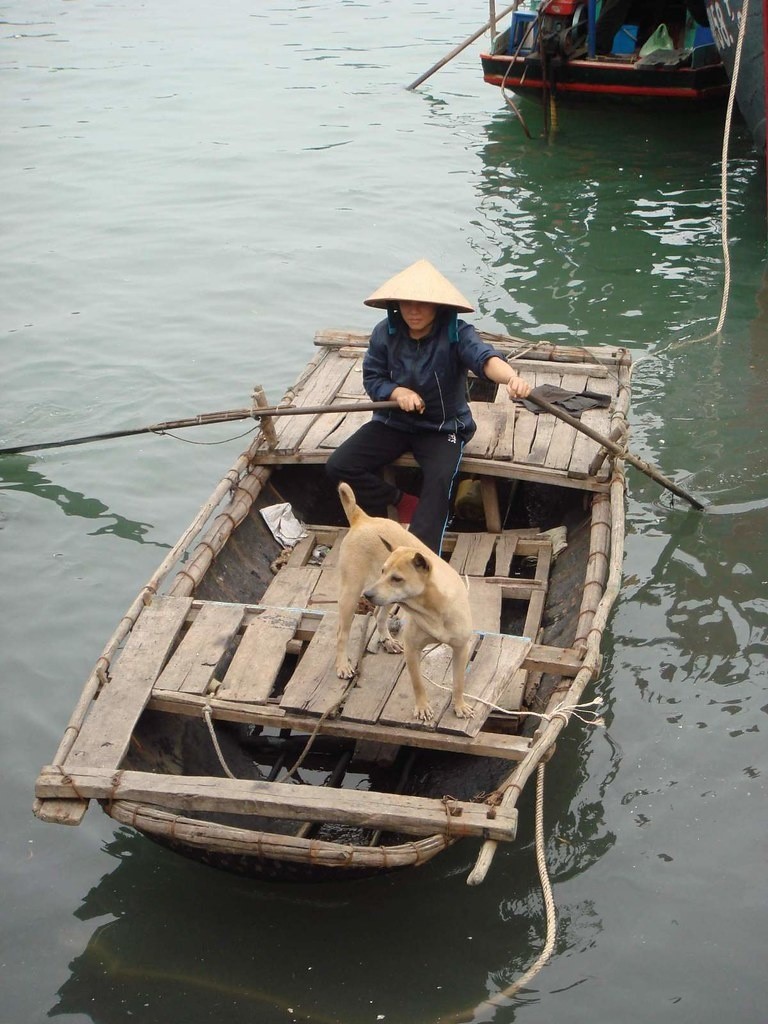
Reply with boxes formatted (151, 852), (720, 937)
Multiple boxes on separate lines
(610, 24), (638, 55)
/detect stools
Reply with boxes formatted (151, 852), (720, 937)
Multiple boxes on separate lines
(507, 11), (538, 55)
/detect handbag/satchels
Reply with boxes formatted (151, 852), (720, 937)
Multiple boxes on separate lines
(639, 23), (673, 58)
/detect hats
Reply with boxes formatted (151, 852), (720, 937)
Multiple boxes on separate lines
(364, 260), (475, 313)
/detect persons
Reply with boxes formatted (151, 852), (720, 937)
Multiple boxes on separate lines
(583, 0), (634, 55)
(324, 259), (533, 558)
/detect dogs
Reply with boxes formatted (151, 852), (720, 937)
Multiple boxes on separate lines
(333, 482), (477, 723)
